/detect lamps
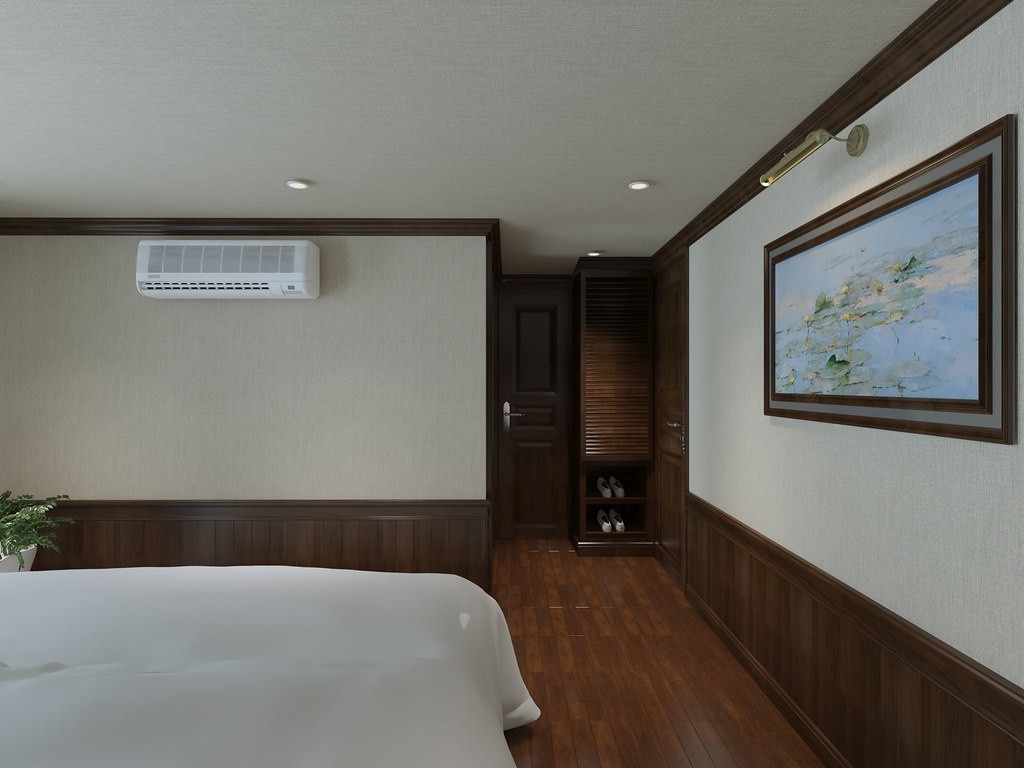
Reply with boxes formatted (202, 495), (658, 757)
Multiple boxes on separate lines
(760, 124), (870, 186)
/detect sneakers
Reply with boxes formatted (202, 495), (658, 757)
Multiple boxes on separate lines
(609, 509), (625, 532)
(609, 476), (625, 497)
(597, 477), (612, 497)
(597, 510), (612, 532)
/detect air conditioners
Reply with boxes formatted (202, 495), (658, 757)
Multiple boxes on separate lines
(135, 240), (320, 300)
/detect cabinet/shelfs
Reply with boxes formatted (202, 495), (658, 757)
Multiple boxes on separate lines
(570, 460), (654, 555)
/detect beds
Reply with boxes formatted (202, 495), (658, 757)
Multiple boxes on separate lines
(0, 565), (540, 768)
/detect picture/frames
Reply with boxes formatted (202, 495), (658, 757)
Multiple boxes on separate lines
(762, 113), (1019, 447)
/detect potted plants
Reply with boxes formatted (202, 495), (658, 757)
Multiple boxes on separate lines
(0, 490), (76, 573)
